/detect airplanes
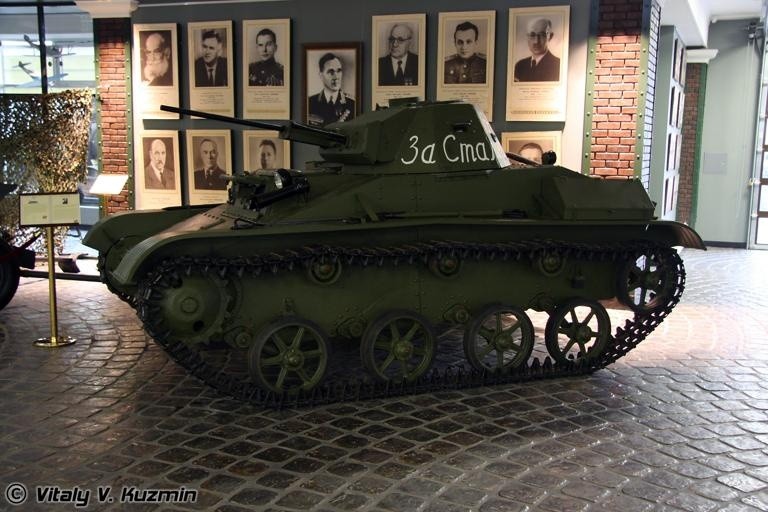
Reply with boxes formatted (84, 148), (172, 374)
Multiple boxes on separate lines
(20, 32), (77, 58)
(10, 60), (70, 89)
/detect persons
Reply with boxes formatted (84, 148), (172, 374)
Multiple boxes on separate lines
(131, 6), (572, 211)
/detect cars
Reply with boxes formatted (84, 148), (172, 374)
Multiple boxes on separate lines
(79, 160), (101, 227)
(0, 181), (40, 317)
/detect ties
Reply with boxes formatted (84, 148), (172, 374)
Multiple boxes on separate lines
(208, 69), (214, 83)
(397, 61), (403, 80)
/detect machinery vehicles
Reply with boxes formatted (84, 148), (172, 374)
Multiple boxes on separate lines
(80, 93), (707, 412)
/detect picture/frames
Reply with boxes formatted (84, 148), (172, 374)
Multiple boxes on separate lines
(301, 41), (362, 127)
